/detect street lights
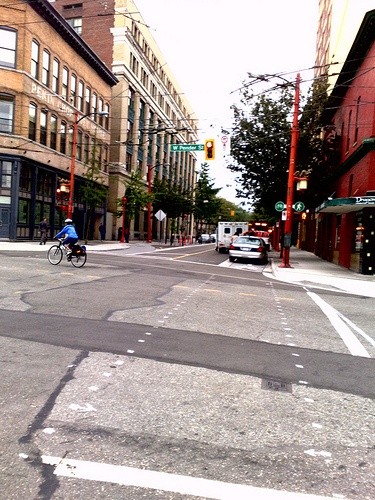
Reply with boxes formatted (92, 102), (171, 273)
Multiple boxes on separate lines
(147, 162), (171, 243)
(257, 71), (309, 268)
(59, 109), (110, 219)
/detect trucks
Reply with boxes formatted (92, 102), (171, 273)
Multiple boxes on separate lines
(216, 221), (249, 255)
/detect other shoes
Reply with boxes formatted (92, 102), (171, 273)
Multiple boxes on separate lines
(66, 250), (72, 257)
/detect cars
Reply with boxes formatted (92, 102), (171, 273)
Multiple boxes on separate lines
(228, 236), (269, 265)
(209, 236), (216, 243)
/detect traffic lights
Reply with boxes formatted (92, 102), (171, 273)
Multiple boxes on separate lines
(203, 138), (215, 160)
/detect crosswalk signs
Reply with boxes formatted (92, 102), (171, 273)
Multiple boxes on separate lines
(294, 202), (305, 212)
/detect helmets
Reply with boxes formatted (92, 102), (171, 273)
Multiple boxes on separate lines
(65, 219), (72, 222)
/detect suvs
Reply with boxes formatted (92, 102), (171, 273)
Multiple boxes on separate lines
(200, 233), (211, 244)
(210, 233), (217, 240)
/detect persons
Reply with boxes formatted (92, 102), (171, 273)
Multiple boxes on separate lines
(53, 219), (78, 261)
(99, 222), (105, 240)
(39, 217), (49, 245)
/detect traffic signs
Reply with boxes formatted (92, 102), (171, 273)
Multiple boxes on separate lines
(170, 144), (204, 152)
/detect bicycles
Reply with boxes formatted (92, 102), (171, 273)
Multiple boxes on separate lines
(47, 237), (87, 268)
